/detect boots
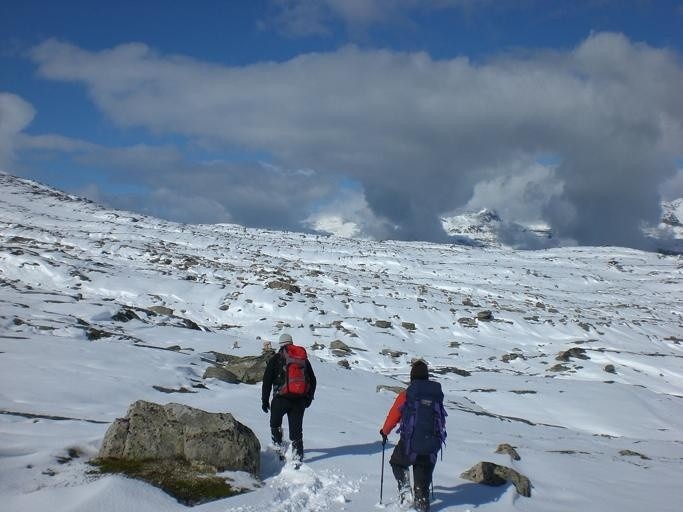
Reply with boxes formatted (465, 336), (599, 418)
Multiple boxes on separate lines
(400, 488), (431, 511)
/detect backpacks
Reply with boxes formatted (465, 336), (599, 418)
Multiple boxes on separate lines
(399, 379), (445, 461)
(273, 345), (310, 397)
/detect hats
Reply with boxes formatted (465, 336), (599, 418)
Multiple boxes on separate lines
(278, 333), (293, 346)
(410, 361), (429, 380)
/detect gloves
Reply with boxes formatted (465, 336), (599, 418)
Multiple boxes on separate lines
(261, 399), (271, 413)
(305, 399), (312, 407)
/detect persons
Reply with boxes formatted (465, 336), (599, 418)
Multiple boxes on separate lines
(380, 360), (445, 512)
(262, 333), (317, 470)
